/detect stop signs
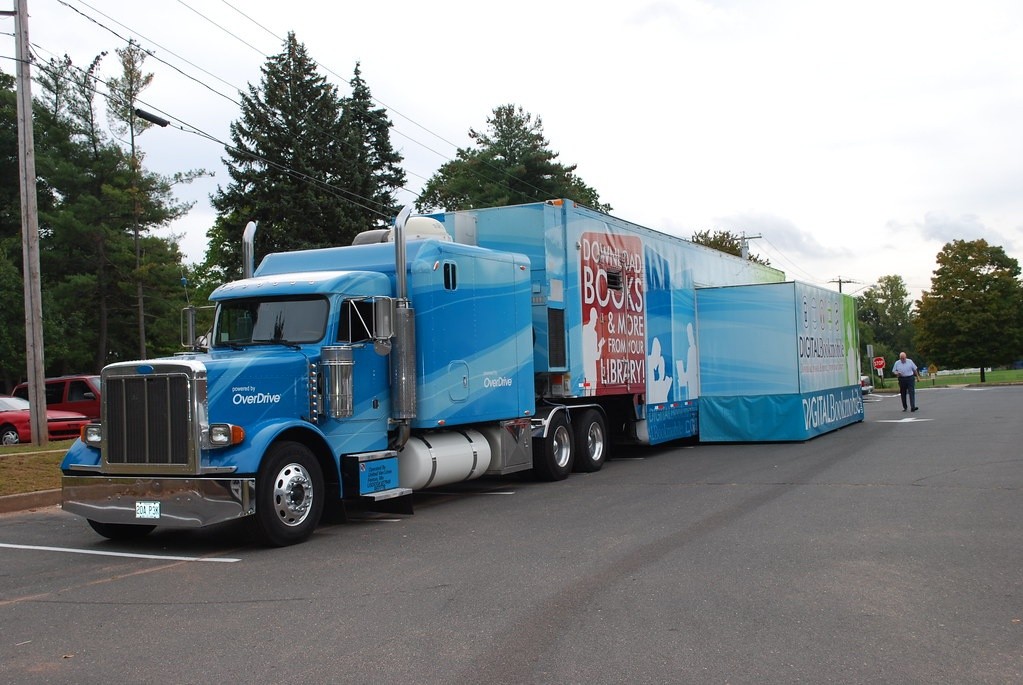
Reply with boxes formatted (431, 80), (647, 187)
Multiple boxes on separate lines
(873, 356), (885, 369)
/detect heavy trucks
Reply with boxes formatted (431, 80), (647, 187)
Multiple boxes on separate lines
(61, 197), (787, 547)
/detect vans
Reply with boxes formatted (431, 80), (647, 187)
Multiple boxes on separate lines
(11, 375), (104, 426)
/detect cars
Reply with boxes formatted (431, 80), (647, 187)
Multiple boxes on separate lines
(860, 376), (875, 395)
(0, 393), (92, 446)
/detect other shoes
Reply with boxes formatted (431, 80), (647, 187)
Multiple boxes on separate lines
(911, 407), (915, 412)
(902, 408), (906, 411)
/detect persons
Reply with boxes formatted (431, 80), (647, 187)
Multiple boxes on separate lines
(892, 352), (920, 412)
